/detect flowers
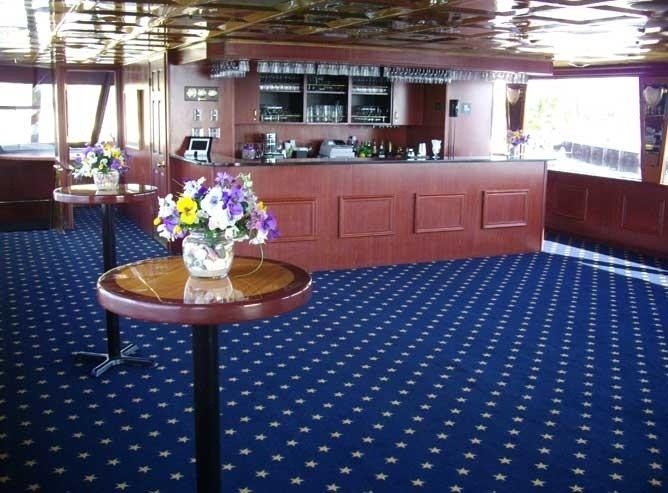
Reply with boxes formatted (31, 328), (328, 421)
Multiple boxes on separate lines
(154, 170), (283, 246)
(70, 139), (132, 179)
(507, 128), (530, 144)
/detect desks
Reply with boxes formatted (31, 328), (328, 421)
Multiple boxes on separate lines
(96, 257), (312, 493)
(54, 183), (159, 379)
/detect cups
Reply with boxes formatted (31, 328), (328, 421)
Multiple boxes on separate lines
(238, 58), (381, 77)
(306, 104), (344, 123)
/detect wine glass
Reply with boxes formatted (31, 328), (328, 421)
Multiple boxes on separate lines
(209, 60), (246, 80)
(350, 78), (388, 94)
(258, 77), (301, 92)
(385, 60), (528, 86)
(431, 140), (442, 160)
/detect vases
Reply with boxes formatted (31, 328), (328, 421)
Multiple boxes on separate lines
(182, 230), (236, 279)
(510, 144), (523, 158)
(94, 171), (119, 190)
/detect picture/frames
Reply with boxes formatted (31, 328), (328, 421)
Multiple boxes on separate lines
(187, 136), (213, 156)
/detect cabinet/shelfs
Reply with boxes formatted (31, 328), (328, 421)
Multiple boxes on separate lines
(258, 64), (395, 128)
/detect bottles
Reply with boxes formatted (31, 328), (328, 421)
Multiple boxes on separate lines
(352, 137), (414, 161)
